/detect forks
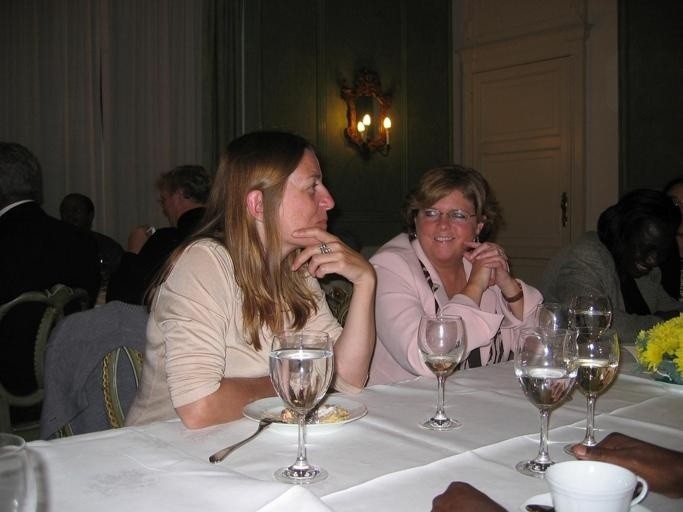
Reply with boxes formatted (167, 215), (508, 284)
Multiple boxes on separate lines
(208, 416), (285, 464)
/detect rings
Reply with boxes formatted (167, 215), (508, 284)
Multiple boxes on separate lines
(319, 243), (331, 254)
(500, 252), (504, 256)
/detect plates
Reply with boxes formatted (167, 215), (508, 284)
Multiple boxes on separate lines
(519, 493), (653, 512)
(240, 394), (369, 427)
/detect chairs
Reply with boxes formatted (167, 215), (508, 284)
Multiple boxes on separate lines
(1, 282), (88, 435)
(36, 300), (148, 440)
(319, 282), (353, 329)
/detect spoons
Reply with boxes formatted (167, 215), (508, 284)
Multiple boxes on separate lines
(525, 504), (554, 512)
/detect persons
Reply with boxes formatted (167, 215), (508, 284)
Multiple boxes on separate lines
(365, 163), (544, 388)
(127, 129), (378, 431)
(59, 192), (122, 250)
(114, 164), (214, 306)
(541, 176), (683, 348)
(0, 141), (125, 430)
(430, 431), (683, 512)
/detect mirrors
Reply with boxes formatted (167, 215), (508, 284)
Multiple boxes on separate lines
(339, 70), (395, 156)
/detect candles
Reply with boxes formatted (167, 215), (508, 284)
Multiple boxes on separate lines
(358, 121), (364, 141)
(362, 114), (370, 143)
(382, 119), (391, 144)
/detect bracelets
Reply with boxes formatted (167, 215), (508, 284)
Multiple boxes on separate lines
(501, 284), (523, 303)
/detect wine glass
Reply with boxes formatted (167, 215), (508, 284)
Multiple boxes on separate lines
(267, 327), (336, 487)
(512, 326), (580, 480)
(415, 312), (468, 433)
(560, 327), (619, 458)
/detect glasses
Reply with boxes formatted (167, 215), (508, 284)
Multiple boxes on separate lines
(423, 208), (477, 223)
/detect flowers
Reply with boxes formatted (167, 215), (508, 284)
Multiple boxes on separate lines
(633, 306), (683, 380)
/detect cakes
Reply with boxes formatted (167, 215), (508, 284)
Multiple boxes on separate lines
(280, 403), (350, 424)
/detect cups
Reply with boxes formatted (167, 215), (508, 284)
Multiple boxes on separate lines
(0, 431), (49, 512)
(532, 293), (613, 333)
(543, 460), (648, 512)
